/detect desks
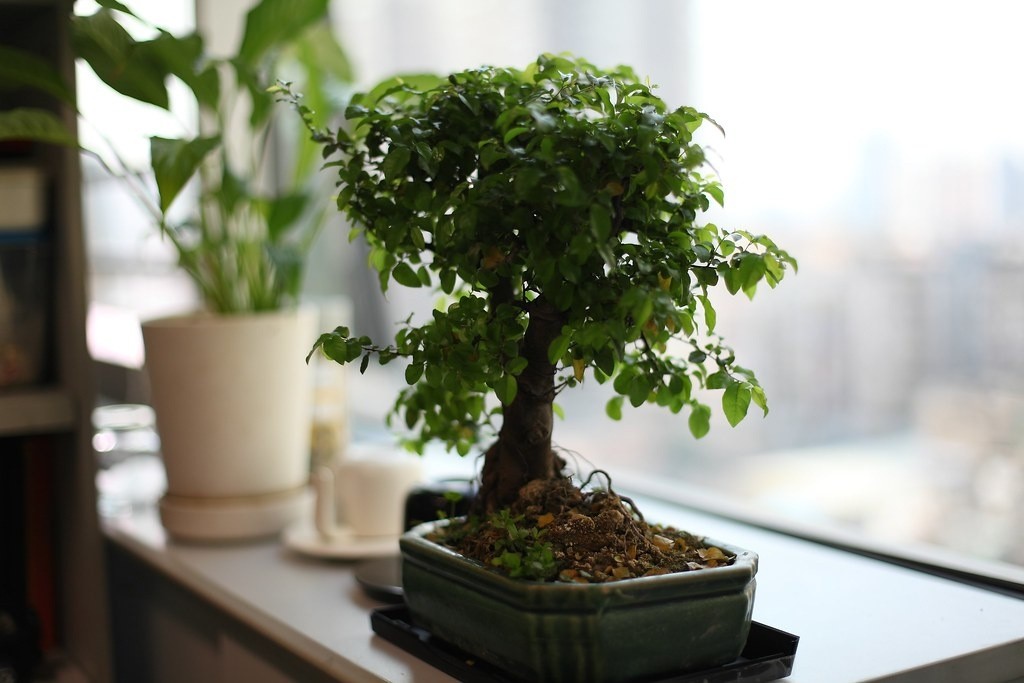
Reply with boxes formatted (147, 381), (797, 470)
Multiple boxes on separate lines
(96, 436), (1024, 683)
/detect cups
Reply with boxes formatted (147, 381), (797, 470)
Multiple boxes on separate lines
(317, 442), (423, 537)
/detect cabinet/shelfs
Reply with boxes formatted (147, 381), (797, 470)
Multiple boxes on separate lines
(0, 0), (112, 683)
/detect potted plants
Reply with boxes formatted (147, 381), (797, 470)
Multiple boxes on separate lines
(0, 0), (362, 546)
(304, 50), (799, 683)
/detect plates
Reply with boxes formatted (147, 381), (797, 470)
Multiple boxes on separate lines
(280, 524), (401, 560)
